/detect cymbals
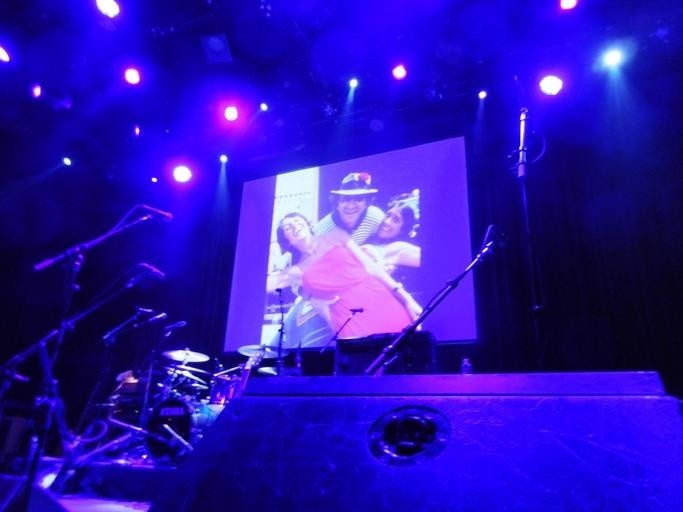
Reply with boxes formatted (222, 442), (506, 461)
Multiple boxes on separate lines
(236, 345), (277, 358)
(163, 368), (206, 384)
(170, 364), (210, 376)
(258, 367), (278, 377)
(160, 350), (210, 364)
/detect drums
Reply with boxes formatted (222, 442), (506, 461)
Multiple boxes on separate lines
(144, 395), (222, 462)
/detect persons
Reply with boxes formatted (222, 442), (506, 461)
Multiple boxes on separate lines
(356, 187), (421, 274)
(273, 168), (386, 347)
(268, 210), (424, 339)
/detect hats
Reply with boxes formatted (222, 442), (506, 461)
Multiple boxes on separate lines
(330, 172), (378, 195)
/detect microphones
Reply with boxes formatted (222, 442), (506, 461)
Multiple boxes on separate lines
(476, 222), (498, 265)
(275, 287), (284, 292)
(133, 312), (170, 331)
(142, 203), (175, 223)
(518, 106), (530, 183)
(141, 263), (168, 279)
(351, 307), (364, 313)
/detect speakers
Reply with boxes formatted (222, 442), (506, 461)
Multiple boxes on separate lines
(148, 370), (683, 512)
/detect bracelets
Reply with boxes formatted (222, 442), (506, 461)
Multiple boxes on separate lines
(391, 280), (404, 292)
